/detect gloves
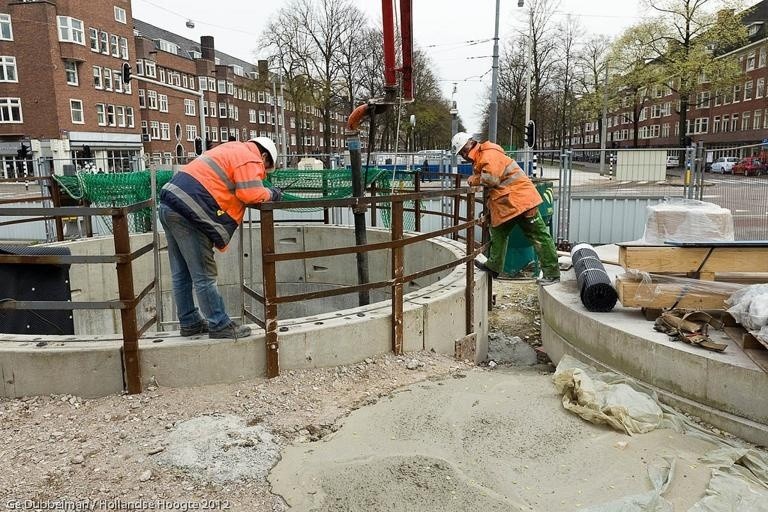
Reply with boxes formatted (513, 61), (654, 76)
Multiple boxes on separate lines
(271, 185), (281, 201)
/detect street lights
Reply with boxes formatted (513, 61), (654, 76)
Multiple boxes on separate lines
(449, 107), (459, 185)
(487, 0), (524, 144)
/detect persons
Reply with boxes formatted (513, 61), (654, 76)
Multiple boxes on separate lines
(97, 167), (104, 173)
(158, 137), (282, 342)
(421, 160), (431, 182)
(451, 131), (561, 286)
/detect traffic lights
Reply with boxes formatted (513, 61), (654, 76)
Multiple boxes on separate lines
(521, 121), (534, 147)
(122, 62), (133, 84)
(17, 143), (23, 160)
(21, 144), (29, 157)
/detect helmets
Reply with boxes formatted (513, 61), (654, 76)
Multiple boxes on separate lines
(451, 131), (472, 155)
(249, 136), (279, 174)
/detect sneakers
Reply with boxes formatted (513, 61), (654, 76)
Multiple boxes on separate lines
(537, 276), (559, 285)
(474, 259), (499, 279)
(208, 322), (251, 340)
(180, 319), (208, 336)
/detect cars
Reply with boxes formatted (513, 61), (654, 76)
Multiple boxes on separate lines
(571, 153), (617, 164)
(731, 157), (767, 178)
(666, 156), (679, 168)
(537, 150), (560, 159)
(709, 156), (738, 174)
